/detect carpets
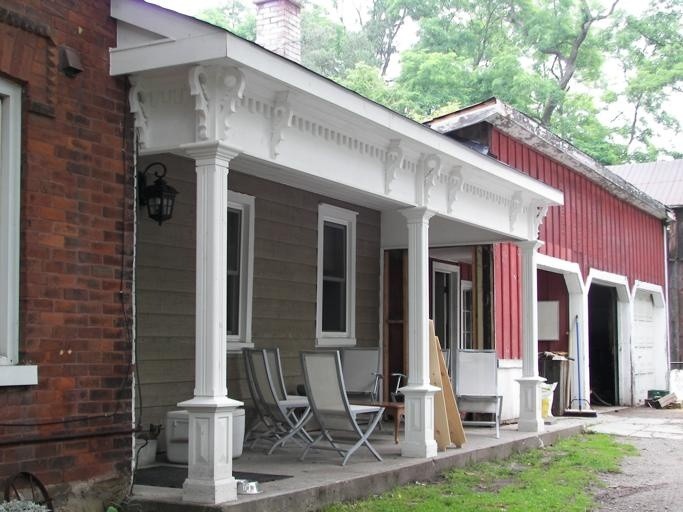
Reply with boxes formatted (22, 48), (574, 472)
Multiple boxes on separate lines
(134, 465), (293, 488)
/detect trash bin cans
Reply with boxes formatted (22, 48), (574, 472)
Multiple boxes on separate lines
(539, 360), (569, 416)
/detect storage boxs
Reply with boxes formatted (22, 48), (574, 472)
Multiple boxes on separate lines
(165, 409), (245, 464)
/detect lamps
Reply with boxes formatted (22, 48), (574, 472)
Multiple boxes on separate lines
(137, 162), (178, 227)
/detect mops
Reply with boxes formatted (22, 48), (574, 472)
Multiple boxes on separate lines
(564, 315), (598, 417)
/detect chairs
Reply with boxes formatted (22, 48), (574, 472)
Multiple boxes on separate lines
(241, 347), (503, 466)
(4, 471), (54, 511)
